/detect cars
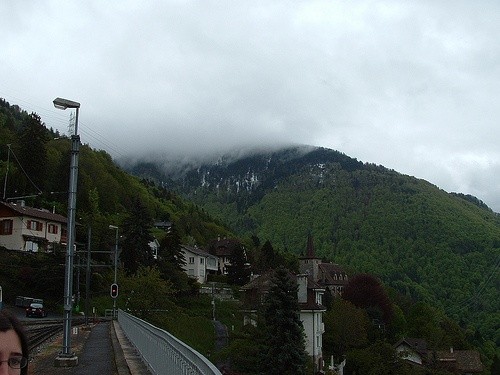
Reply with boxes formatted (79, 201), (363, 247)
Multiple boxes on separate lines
(23, 298), (48, 318)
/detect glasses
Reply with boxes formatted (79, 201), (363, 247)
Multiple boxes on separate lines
(0, 356), (31, 369)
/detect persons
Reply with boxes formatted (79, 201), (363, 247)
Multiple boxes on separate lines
(0, 310), (29, 375)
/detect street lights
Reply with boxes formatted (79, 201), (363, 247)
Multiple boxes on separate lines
(53, 97), (82, 358)
(108, 225), (118, 320)
